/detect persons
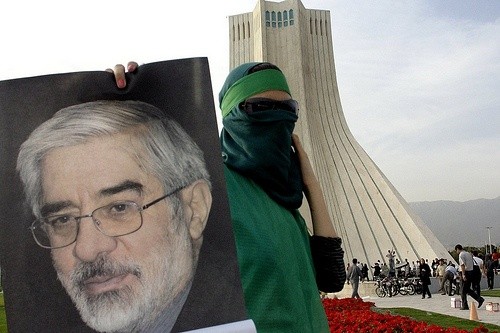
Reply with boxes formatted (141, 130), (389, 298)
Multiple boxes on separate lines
(419, 258), (432, 299)
(396, 259), (428, 278)
(436, 260), (447, 295)
(357, 261), (369, 282)
(484, 254), (496, 290)
(347, 263), (351, 285)
(16, 101), (213, 333)
(455, 244), (485, 310)
(471, 252), (483, 296)
(106, 61), (346, 333)
(492, 249), (499, 269)
(485, 250), (492, 269)
(426, 258), (455, 277)
(438, 264), (458, 296)
(371, 260), (387, 281)
(346, 258), (363, 298)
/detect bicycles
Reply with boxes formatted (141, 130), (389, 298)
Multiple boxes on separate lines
(374, 274), (423, 298)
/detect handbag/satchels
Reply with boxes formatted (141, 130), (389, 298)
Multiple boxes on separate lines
(472, 259), (482, 285)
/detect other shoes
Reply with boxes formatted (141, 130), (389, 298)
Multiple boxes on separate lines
(478, 297), (484, 308)
(460, 307), (469, 310)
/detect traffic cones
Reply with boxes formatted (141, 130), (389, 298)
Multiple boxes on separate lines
(469, 301), (481, 321)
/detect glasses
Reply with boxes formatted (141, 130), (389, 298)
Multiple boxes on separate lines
(26, 186), (186, 248)
(240, 97), (296, 114)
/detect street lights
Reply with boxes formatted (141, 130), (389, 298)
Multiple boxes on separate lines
(486, 226), (493, 254)
